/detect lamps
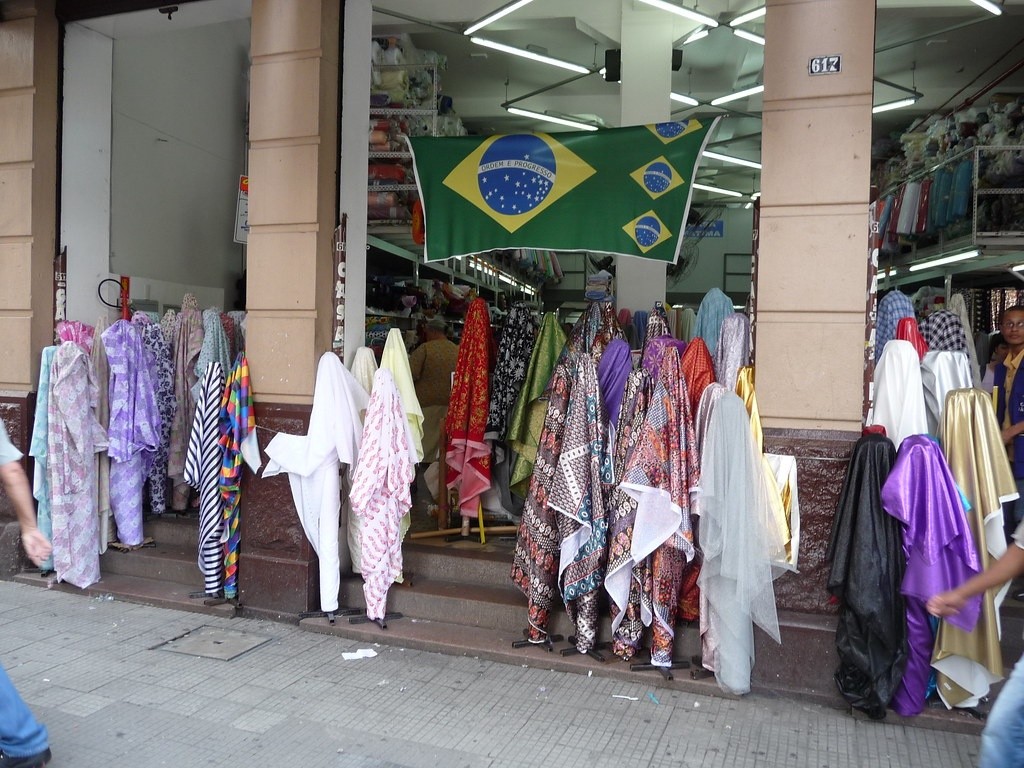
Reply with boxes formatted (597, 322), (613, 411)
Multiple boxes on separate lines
(732, 28), (765, 45)
(500, 79), (600, 132)
(640, 0), (718, 28)
(693, 183), (744, 197)
(711, 84), (765, 107)
(702, 150), (761, 170)
(467, 35), (591, 76)
(873, 62), (924, 114)
(730, 4), (768, 27)
(909, 248), (983, 273)
(462, 0), (534, 36)
(669, 91), (700, 107)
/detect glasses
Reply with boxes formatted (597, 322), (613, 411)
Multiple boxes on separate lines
(1000, 319), (1024, 328)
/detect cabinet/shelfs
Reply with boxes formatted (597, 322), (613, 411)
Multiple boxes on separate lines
(365, 35), (564, 363)
(869, 95), (1024, 294)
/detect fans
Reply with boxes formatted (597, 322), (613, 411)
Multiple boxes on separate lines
(666, 243), (699, 290)
(683, 198), (728, 245)
(583, 253), (617, 277)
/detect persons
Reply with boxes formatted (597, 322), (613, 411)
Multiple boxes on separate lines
(0, 414), (51, 768)
(971, 306), (1024, 602)
(408, 319), (461, 514)
(924, 513), (1024, 768)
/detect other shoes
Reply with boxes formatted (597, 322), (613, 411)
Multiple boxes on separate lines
(0, 747), (51, 768)
